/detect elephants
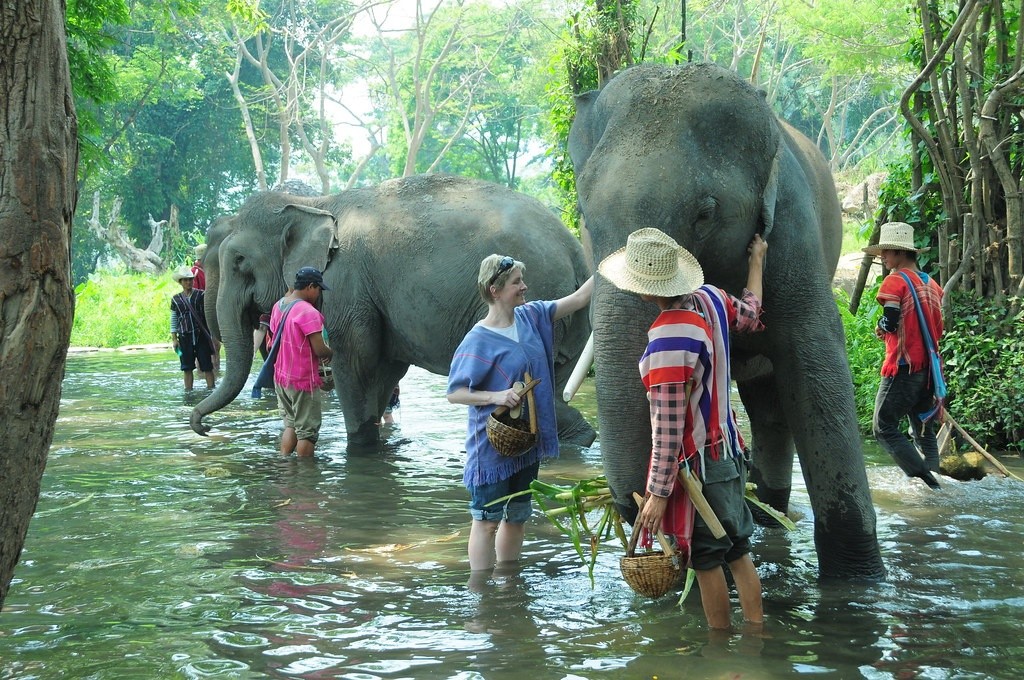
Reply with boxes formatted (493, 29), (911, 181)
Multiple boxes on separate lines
(188, 173), (598, 448)
(563, 64), (889, 585)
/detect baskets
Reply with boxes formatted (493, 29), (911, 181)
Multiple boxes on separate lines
(486, 371), (541, 457)
(619, 492), (682, 599)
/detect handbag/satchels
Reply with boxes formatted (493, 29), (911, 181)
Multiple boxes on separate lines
(932, 356), (946, 401)
(641, 462), (700, 568)
(207, 334), (216, 356)
(251, 352), (277, 398)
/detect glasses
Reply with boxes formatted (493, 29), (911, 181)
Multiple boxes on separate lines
(490, 256), (513, 285)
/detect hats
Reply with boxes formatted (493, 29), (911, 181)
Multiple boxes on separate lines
(597, 227), (704, 297)
(295, 266), (330, 290)
(861, 222), (931, 255)
(195, 244), (208, 260)
(171, 265), (198, 282)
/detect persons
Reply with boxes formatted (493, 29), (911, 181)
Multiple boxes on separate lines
(170, 266), (220, 387)
(270, 267), (333, 456)
(597, 228), (768, 629)
(447, 253), (594, 571)
(190, 244), (221, 371)
(383, 382), (400, 423)
(861, 223), (948, 488)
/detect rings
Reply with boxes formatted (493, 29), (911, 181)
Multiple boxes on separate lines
(649, 521), (653, 524)
